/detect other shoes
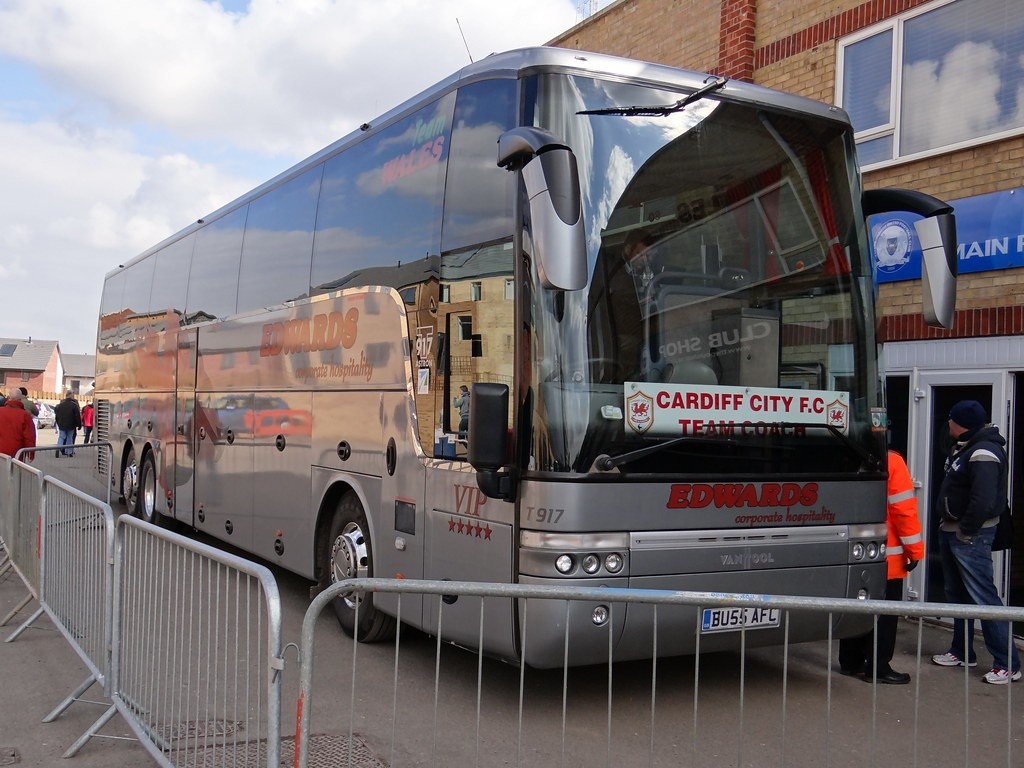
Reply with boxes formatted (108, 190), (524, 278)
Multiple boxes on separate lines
(67, 454), (73, 457)
(62, 451), (66, 455)
(55, 450), (59, 458)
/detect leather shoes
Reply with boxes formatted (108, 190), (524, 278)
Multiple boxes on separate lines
(864, 669), (910, 684)
(840, 666), (865, 675)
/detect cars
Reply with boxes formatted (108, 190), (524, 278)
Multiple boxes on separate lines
(114, 391), (313, 442)
(30, 403), (59, 429)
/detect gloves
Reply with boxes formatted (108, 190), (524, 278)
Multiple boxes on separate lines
(904, 560), (918, 571)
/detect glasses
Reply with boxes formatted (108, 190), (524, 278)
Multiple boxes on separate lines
(947, 416), (952, 421)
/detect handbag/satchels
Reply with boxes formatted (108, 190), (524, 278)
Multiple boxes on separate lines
(991, 506), (1015, 551)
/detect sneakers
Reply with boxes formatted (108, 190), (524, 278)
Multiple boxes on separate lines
(983, 668), (1022, 684)
(931, 652), (978, 667)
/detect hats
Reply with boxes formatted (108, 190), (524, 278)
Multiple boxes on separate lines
(950, 400), (986, 429)
(19, 387), (27, 395)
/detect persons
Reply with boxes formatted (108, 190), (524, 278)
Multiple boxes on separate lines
(53, 391), (94, 457)
(453, 385), (471, 442)
(607, 228), (657, 306)
(932, 402), (1023, 684)
(839, 449), (924, 685)
(0, 386), (39, 464)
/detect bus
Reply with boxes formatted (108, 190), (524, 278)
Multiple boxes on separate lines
(93, 46), (956, 672)
